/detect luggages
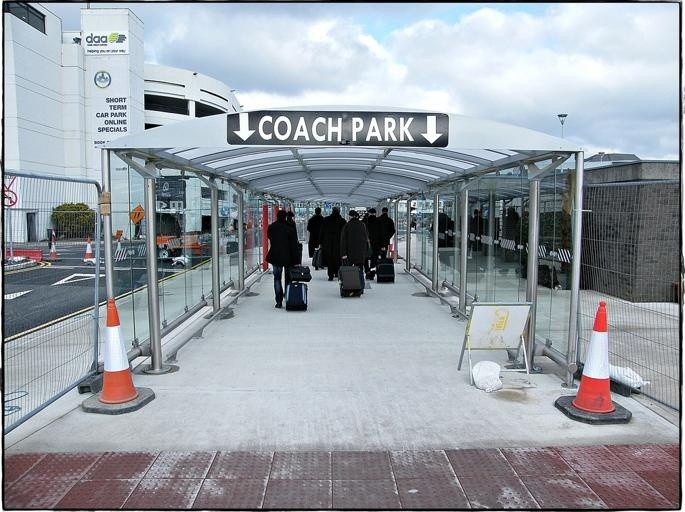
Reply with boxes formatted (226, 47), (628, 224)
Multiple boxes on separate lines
(337, 256), (364, 298)
(284, 282), (308, 313)
(377, 257), (395, 284)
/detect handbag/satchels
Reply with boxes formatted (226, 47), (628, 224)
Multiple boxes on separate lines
(311, 249), (326, 270)
(288, 265), (313, 282)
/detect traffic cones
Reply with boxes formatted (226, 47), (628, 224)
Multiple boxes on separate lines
(84, 237), (94, 258)
(49, 242), (58, 258)
(82, 299), (155, 414)
(555, 300), (632, 425)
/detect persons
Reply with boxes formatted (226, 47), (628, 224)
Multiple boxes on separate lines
(286, 210), (300, 245)
(266, 210), (302, 309)
(305, 204), (396, 295)
(411, 204), (529, 274)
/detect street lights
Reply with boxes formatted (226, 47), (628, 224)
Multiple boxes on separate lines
(558, 113), (568, 137)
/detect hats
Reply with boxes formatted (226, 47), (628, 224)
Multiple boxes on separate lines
(368, 207), (377, 213)
(381, 207), (388, 213)
(348, 210), (357, 216)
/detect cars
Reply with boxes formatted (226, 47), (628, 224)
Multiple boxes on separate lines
(157, 231), (208, 262)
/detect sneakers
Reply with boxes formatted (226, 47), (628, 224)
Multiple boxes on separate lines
(275, 302), (282, 308)
